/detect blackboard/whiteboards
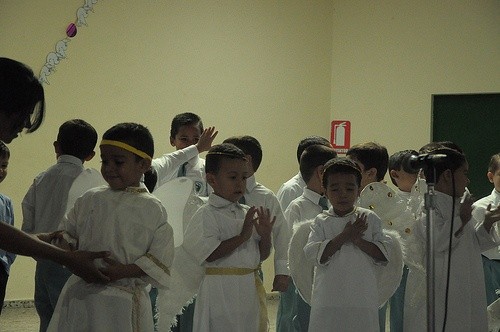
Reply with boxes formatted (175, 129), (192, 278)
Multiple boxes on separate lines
(429, 92), (500, 205)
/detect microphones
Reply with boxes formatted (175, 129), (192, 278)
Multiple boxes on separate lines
(403, 153), (448, 173)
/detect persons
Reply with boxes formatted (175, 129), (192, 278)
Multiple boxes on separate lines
(182, 144), (276, 332)
(472, 153), (500, 308)
(0, 142), (17, 318)
(20, 119), (219, 332)
(142, 113), (471, 332)
(403, 148), (500, 332)
(303, 157), (392, 332)
(0, 56), (112, 332)
(45, 123), (175, 332)
